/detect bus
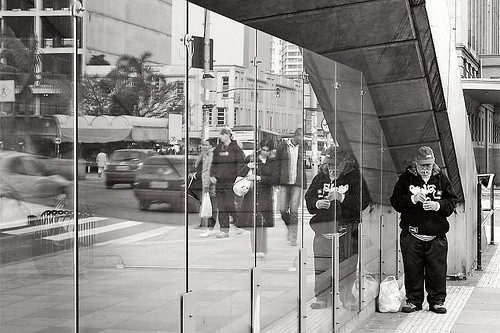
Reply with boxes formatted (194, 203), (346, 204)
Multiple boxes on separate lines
(233, 127), (326, 169)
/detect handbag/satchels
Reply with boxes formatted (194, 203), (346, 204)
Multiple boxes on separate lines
(352, 276), (379, 307)
(199, 192), (213, 219)
(233, 155), (252, 196)
(378, 276), (403, 313)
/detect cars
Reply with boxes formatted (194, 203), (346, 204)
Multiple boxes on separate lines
(0, 151), (71, 203)
(105, 149), (156, 188)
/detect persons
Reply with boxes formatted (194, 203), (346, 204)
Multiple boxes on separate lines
(276, 128), (307, 246)
(389, 146), (457, 313)
(167, 145), (185, 155)
(191, 140), (216, 230)
(95, 149), (107, 178)
(209, 127), (247, 238)
(239, 138), (279, 258)
(305, 146), (371, 311)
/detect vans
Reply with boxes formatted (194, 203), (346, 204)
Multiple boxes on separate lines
(132, 155), (202, 212)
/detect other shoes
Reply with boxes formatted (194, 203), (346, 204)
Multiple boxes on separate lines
(237, 228), (243, 234)
(194, 226), (207, 229)
(290, 238), (296, 246)
(200, 229), (214, 237)
(216, 231), (229, 238)
(255, 251), (264, 258)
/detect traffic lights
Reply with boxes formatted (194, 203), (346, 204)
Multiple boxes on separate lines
(276, 88), (280, 98)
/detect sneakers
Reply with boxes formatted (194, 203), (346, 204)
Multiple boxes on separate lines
(403, 302), (417, 313)
(340, 292), (360, 310)
(310, 299), (331, 308)
(429, 303), (446, 313)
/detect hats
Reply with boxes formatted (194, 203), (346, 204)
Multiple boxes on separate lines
(417, 145), (435, 165)
(324, 146), (343, 165)
